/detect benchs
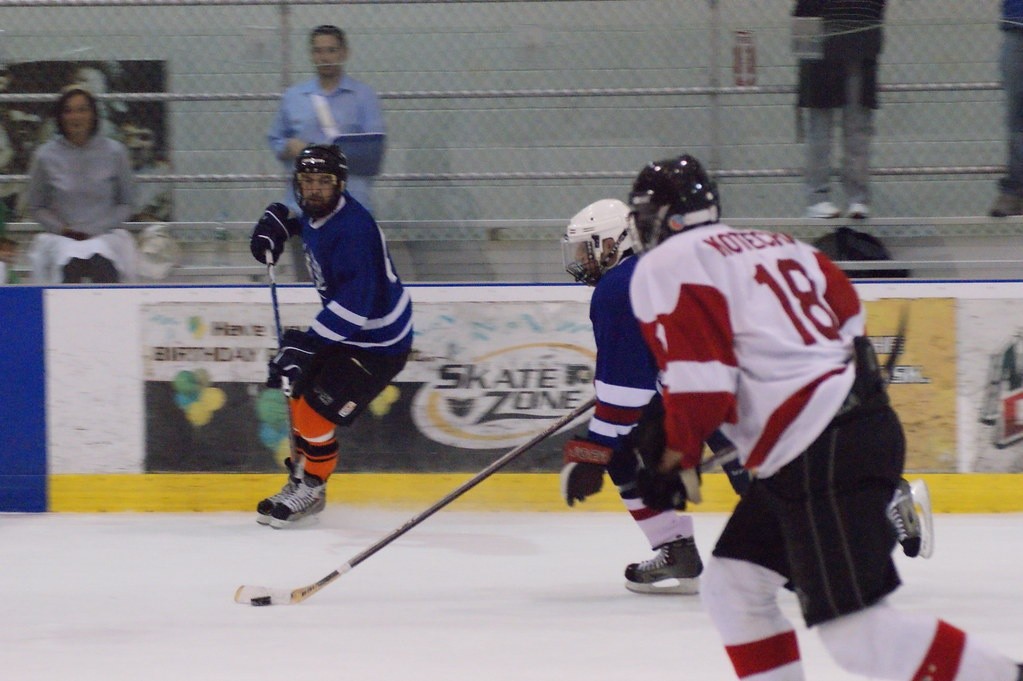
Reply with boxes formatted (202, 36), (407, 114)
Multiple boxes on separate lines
(2, 215), (1023, 275)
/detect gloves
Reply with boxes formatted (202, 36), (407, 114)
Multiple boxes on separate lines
(250, 204), (294, 264)
(266, 328), (321, 390)
(638, 458), (702, 512)
(562, 434), (613, 507)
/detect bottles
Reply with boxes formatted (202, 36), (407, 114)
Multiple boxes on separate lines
(217, 229), (230, 268)
(733, 31), (757, 87)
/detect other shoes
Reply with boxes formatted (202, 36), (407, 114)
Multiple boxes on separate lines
(990, 192), (1023, 217)
(804, 201), (840, 218)
(848, 201), (871, 220)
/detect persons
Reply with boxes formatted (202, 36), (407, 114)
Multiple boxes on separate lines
(987, 0), (1023, 217)
(563, 154), (1023, 681)
(26, 85), (133, 286)
(267, 25), (386, 282)
(251, 143), (412, 531)
(791, 0), (885, 219)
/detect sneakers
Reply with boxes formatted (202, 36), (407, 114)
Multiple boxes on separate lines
(255, 457), (305, 524)
(271, 471), (330, 529)
(886, 479), (935, 559)
(624, 535), (704, 593)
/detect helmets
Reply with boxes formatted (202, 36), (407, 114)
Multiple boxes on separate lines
(627, 154), (720, 249)
(292, 143), (347, 218)
(566, 199), (635, 266)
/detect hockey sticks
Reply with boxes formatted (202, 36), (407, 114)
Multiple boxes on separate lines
(233, 396), (598, 607)
(265, 249), (303, 483)
